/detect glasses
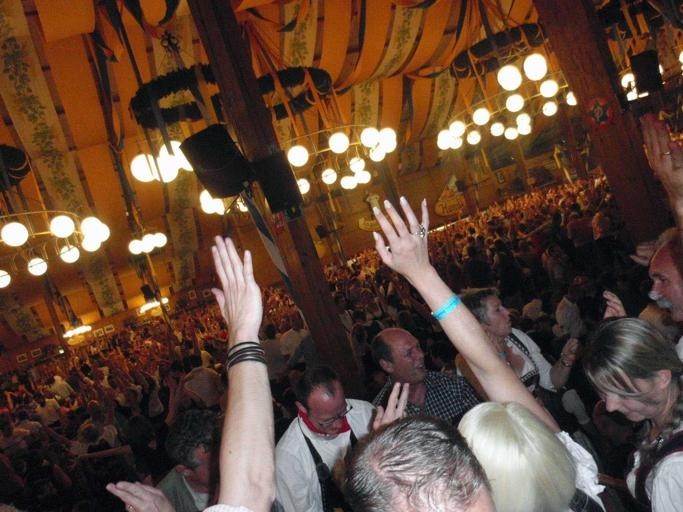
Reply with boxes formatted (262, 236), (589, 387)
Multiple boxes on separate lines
(305, 396), (354, 430)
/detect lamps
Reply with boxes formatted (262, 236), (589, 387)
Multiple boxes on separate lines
(131, 61), (257, 215)
(126, 193), (168, 256)
(0, 143), (113, 290)
(257, 66), (397, 196)
(432, 21), (578, 153)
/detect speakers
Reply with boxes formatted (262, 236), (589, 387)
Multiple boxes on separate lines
(250, 149), (304, 216)
(629, 47), (664, 96)
(179, 122), (256, 200)
